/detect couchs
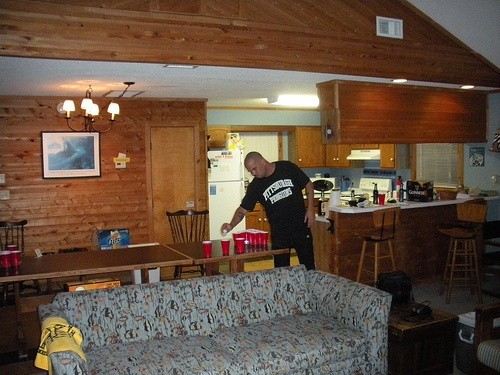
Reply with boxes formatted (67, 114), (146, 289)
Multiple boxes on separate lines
(35, 265), (392, 375)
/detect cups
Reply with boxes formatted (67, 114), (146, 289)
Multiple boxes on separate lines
(378, 194), (385, 206)
(233, 229), (268, 254)
(0, 245), (23, 269)
(221, 239), (230, 256)
(202, 241), (212, 258)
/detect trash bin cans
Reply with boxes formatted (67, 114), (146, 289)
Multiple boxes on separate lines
(376, 271), (412, 303)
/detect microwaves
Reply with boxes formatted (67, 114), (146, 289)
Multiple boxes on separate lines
(310, 177), (339, 198)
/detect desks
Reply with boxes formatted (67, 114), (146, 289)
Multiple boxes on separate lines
(164, 234), (290, 276)
(0, 244), (193, 359)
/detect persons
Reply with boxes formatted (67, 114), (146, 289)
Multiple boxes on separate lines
(222, 152), (315, 270)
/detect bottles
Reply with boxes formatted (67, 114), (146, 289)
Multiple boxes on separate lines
(351, 190), (355, 200)
(318, 189), (327, 216)
(395, 176), (404, 202)
(373, 184), (379, 204)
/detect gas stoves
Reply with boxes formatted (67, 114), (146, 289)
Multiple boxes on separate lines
(339, 178), (392, 197)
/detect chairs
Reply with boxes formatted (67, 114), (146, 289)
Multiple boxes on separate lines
(0, 220), (27, 307)
(473, 302), (500, 375)
(167, 210), (209, 279)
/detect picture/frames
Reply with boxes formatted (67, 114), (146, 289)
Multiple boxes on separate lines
(41, 131), (101, 178)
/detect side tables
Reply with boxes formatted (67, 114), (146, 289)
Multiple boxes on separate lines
(387, 303), (459, 375)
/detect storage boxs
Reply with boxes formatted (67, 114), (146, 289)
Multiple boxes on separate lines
(455, 311), (500, 375)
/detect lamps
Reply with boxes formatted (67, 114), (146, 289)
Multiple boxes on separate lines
(58, 82), (121, 132)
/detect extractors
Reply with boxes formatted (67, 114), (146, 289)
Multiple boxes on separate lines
(346, 149), (381, 161)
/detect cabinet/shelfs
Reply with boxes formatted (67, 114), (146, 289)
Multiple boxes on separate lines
(288, 126), (397, 168)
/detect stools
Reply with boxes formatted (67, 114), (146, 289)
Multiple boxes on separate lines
(353, 206), (401, 288)
(440, 202), (488, 304)
